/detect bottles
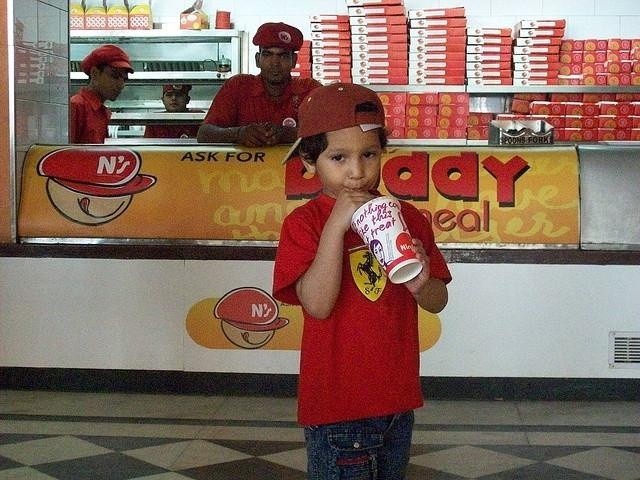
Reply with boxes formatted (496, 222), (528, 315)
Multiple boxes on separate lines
(218, 59), (230, 72)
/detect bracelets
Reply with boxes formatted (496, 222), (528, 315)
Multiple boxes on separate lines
(237, 126), (243, 143)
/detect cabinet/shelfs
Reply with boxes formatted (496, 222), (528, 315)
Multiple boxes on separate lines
(70, 29), (243, 145)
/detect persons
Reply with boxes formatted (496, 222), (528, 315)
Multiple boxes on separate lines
(369, 239), (391, 270)
(270, 81), (452, 479)
(68, 43), (134, 144)
(195, 21), (322, 151)
(141, 83), (208, 139)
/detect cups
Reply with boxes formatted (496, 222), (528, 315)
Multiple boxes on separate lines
(351, 194), (422, 284)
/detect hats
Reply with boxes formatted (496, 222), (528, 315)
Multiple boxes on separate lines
(162, 83), (192, 94)
(252, 22), (304, 52)
(280, 81), (387, 168)
(81, 44), (135, 75)
